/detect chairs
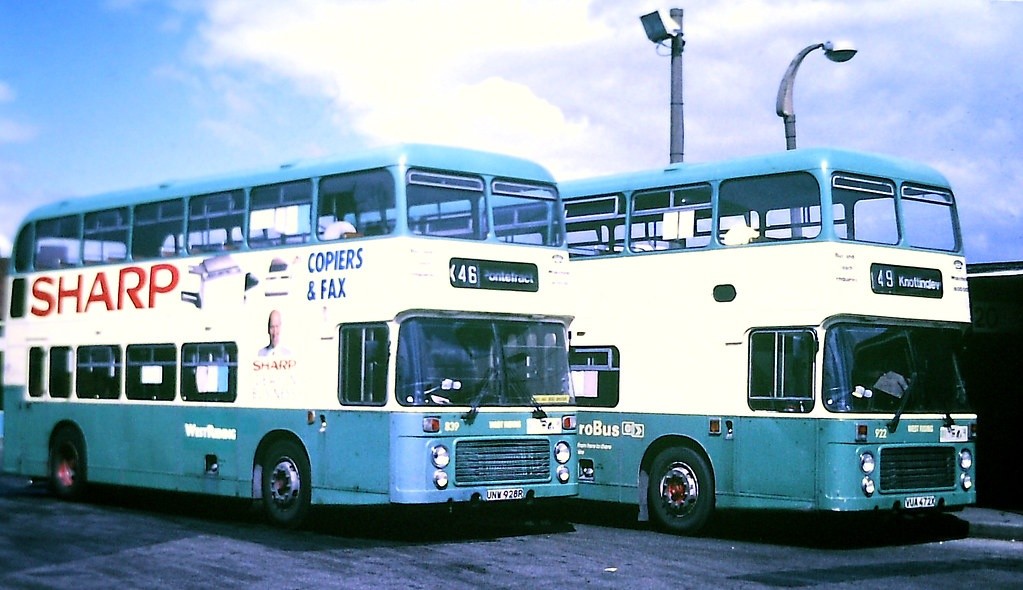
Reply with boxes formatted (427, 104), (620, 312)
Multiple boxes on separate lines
(569, 232), (780, 258)
(32, 376), (235, 402)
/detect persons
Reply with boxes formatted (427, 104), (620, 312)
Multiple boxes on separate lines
(253, 311), (291, 395)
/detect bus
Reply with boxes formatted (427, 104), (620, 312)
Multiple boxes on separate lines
(332, 144), (981, 537)
(0, 146), (588, 530)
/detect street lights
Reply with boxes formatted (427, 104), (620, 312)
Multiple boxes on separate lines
(775, 37), (859, 241)
